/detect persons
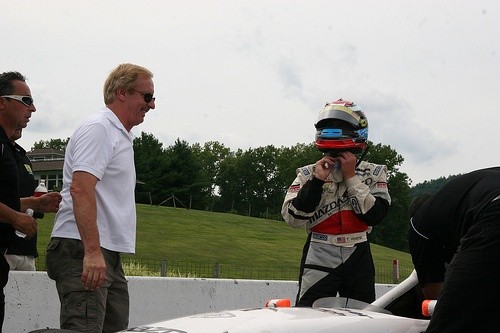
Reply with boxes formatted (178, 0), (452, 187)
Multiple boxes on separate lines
(280, 98), (392, 308)
(409, 166), (500, 333)
(46, 63), (156, 333)
(0, 71), (62, 333)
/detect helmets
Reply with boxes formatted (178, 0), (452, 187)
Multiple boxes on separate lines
(314, 97), (368, 152)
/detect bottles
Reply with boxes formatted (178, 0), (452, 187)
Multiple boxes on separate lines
(15, 208), (34, 238)
(32, 179), (48, 220)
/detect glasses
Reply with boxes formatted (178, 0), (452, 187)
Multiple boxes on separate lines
(0, 94), (33, 105)
(130, 88), (155, 102)
